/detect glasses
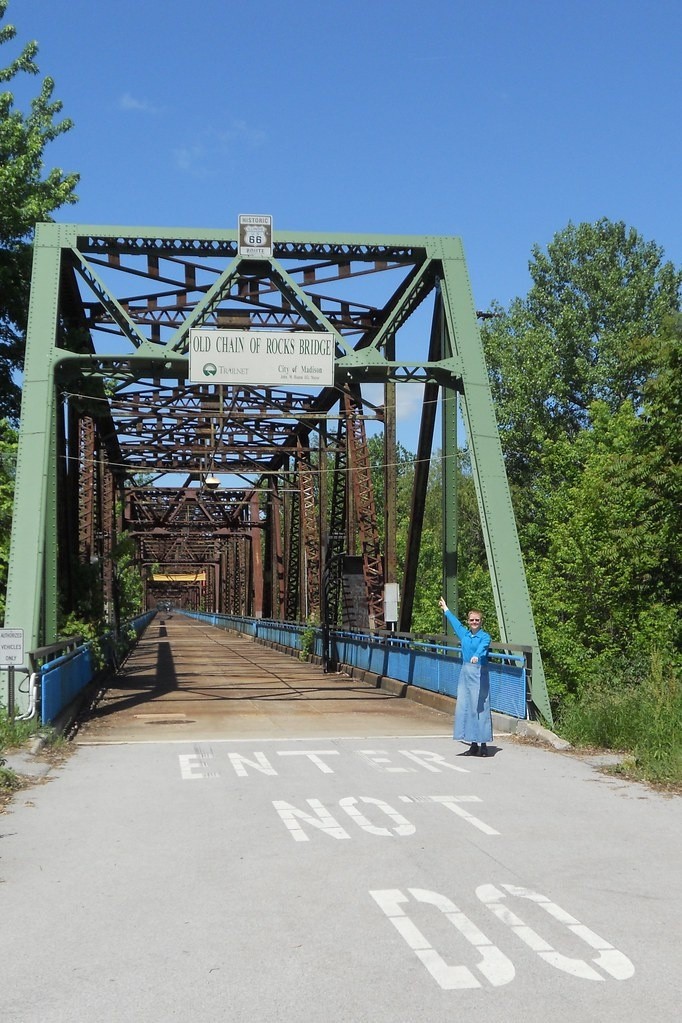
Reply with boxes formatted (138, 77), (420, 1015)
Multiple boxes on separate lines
(470, 619), (480, 621)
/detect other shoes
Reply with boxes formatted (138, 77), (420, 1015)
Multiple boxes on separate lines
(465, 747), (477, 756)
(481, 747), (487, 757)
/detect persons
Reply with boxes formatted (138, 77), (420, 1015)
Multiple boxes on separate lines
(439, 597), (493, 757)
